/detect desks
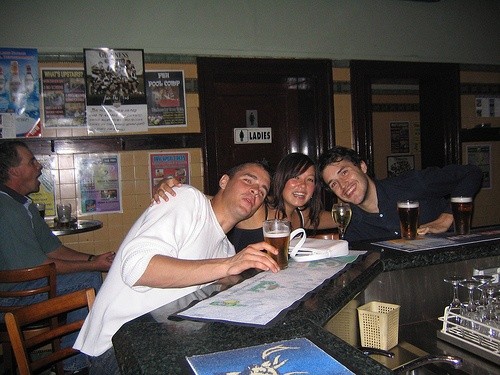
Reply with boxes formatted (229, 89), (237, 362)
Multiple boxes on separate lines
(47, 220), (103, 236)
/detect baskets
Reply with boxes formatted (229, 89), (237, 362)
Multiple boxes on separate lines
(357, 301), (400, 351)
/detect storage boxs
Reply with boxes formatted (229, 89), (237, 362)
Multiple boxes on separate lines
(356, 301), (400, 350)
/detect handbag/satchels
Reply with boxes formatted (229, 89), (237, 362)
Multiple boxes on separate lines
(288, 227), (349, 262)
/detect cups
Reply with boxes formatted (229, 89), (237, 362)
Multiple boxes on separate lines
(451, 195), (473, 236)
(57, 203), (72, 223)
(397, 199), (419, 240)
(35, 204), (45, 220)
(262, 219), (290, 270)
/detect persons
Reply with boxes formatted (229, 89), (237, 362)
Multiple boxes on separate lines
(149, 151), (343, 275)
(318, 145), (483, 244)
(0, 138), (118, 375)
(91, 58), (139, 101)
(71, 160), (281, 375)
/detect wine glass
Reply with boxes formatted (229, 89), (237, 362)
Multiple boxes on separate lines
(444, 275), (500, 340)
(331, 203), (353, 239)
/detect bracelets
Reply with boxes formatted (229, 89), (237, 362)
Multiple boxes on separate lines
(87, 255), (94, 262)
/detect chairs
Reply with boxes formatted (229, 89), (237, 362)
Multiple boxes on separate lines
(0, 263), (96, 375)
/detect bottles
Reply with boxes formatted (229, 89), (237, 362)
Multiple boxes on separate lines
(0, 61), (34, 107)
